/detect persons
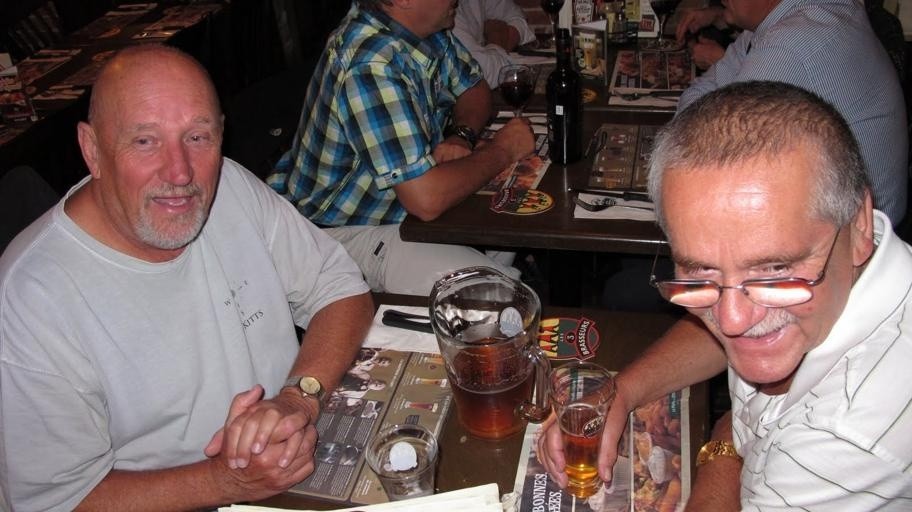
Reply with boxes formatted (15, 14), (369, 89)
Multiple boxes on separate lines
(536, 79), (912, 512)
(672, 4), (737, 70)
(265, 1), (540, 309)
(452, 0), (539, 90)
(673, 0), (912, 238)
(0, 37), (379, 512)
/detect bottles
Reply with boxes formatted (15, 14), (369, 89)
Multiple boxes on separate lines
(547, 29), (583, 164)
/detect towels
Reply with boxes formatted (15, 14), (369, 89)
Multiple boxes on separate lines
(358, 302), (499, 358)
(489, 110), (548, 134)
(509, 48), (557, 66)
(607, 86), (688, 109)
(571, 190), (658, 223)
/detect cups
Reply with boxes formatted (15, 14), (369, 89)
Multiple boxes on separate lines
(640, 0), (684, 51)
(546, 359), (618, 499)
(497, 62), (538, 119)
(538, 0), (566, 49)
(428, 265), (556, 444)
(365, 423), (439, 503)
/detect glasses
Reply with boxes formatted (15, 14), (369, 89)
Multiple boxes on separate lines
(647, 224), (843, 310)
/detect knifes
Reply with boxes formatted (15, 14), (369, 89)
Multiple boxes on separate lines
(568, 188), (654, 203)
(611, 93), (678, 103)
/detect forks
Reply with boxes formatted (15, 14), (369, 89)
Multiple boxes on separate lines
(569, 196), (657, 213)
(383, 309), (492, 327)
(615, 91), (684, 101)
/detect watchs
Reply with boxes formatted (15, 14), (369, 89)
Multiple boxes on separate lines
(279, 374), (328, 410)
(694, 442), (744, 466)
